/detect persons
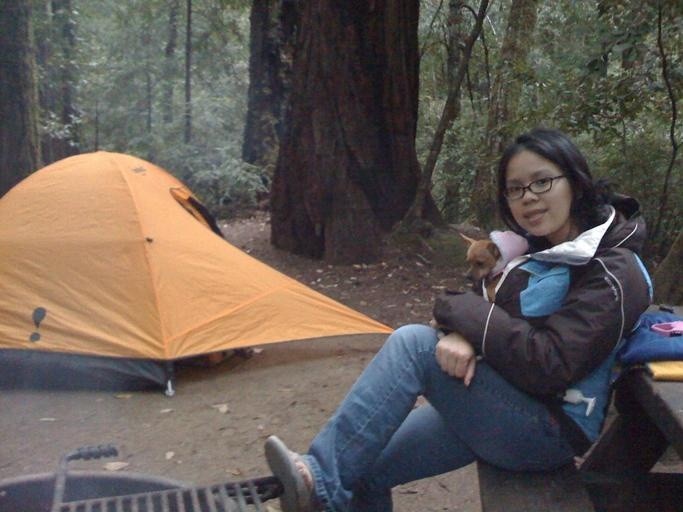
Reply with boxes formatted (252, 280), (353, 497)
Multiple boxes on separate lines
(262, 125), (656, 512)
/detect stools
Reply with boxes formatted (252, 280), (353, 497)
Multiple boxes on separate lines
(476, 461), (594, 512)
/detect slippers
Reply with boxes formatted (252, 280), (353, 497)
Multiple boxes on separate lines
(264, 436), (315, 511)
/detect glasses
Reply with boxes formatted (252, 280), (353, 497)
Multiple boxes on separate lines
(501, 174), (566, 200)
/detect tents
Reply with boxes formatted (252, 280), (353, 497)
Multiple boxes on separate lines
(0, 145), (399, 402)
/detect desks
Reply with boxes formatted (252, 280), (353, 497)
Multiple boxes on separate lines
(620, 305), (683, 460)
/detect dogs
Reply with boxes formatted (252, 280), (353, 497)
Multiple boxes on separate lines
(458, 230), (537, 306)
